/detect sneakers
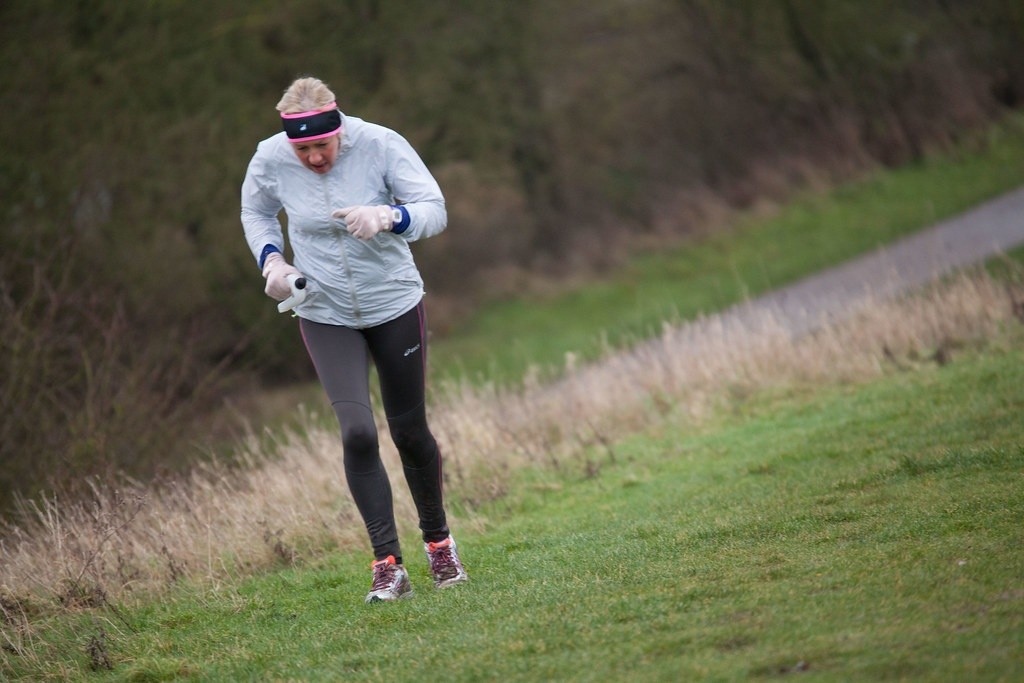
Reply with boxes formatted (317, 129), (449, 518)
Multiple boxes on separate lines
(364, 555), (414, 605)
(424, 534), (467, 589)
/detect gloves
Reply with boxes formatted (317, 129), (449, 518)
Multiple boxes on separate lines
(332, 203), (392, 241)
(262, 252), (303, 300)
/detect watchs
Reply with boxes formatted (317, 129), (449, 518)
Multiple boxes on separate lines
(393, 205), (403, 228)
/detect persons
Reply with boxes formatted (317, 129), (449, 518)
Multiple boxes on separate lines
(241, 74), (466, 604)
